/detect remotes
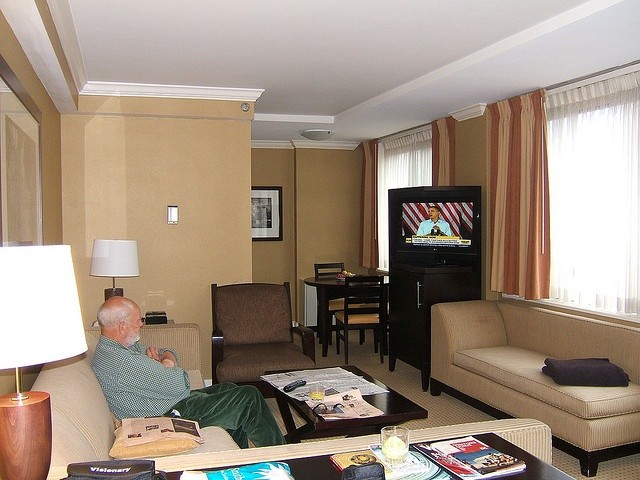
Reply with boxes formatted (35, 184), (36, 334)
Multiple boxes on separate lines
(283, 380), (308, 393)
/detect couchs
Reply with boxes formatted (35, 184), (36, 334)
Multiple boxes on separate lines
(428, 299), (640, 477)
(26, 324), (240, 477)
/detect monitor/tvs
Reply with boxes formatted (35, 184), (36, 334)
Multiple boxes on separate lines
(388, 186), (482, 269)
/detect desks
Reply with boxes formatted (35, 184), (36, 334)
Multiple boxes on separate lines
(126, 433), (577, 478)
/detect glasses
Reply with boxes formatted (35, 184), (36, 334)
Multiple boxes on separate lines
(312, 403), (350, 418)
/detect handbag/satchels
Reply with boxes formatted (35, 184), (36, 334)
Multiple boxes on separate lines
(66, 459), (168, 480)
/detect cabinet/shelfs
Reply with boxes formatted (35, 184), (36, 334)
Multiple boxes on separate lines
(388, 260), (480, 392)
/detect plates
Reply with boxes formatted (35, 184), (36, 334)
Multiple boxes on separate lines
(334, 270), (357, 284)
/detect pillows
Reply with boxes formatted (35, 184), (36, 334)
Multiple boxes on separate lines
(111, 423), (197, 458)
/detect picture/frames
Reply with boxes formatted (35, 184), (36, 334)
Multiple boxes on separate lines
(251, 185), (284, 241)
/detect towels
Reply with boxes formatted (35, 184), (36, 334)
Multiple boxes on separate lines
(542, 357), (631, 387)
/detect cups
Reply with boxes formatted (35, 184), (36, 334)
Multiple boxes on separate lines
(380, 426), (410, 465)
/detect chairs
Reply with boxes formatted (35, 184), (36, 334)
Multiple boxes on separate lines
(211, 281), (317, 398)
(335, 275), (385, 364)
(314, 262), (345, 345)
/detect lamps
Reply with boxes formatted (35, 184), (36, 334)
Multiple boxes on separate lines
(300, 129), (334, 141)
(0, 244), (89, 480)
(89, 238), (139, 301)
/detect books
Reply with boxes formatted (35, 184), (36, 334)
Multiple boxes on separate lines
(330, 445), (456, 480)
(413, 436), (526, 480)
(304, 388), (383, 421)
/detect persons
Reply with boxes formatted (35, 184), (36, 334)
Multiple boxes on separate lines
(92, 297), (288, 448)
(416, 206), (452, 237)
